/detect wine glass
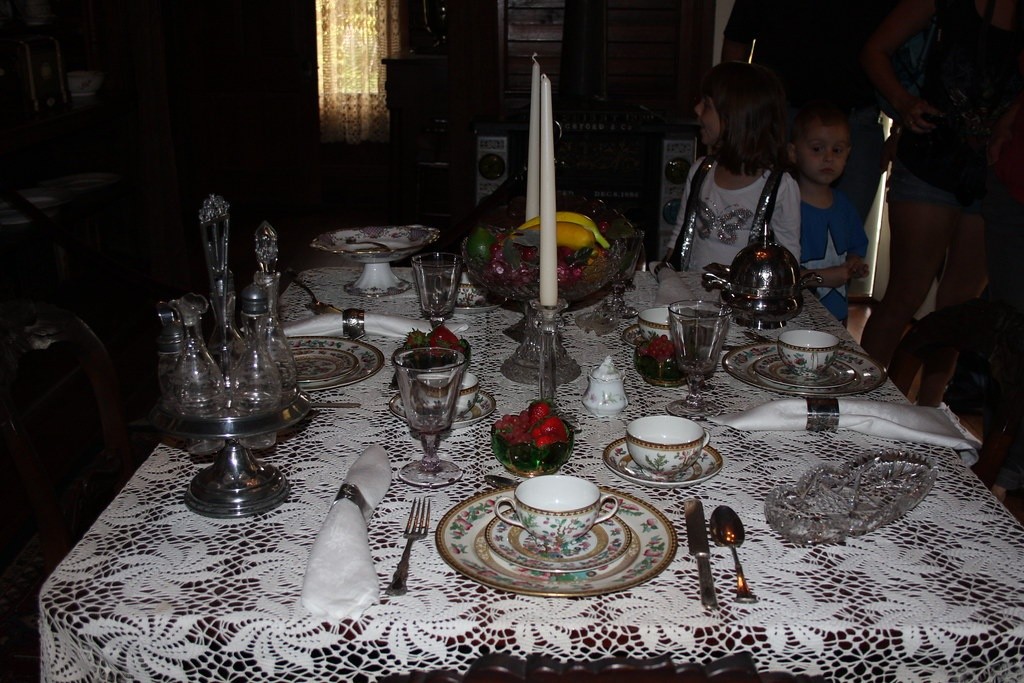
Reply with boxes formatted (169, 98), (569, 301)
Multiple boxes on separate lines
(666, 298), (734, 420)
(395, 347), (468, 487)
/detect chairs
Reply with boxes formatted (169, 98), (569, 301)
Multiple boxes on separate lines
(886, 297), (1024, 490)
(0, 300), (137, 578)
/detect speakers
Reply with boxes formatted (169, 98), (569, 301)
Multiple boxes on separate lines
(657, 132), (698, 261)
(475, 130), (517, 208)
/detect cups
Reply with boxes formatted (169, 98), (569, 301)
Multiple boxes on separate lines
(412, 252), (466, 329)
(494, 475), (620, 544)
(581, 355), (630, 419)
(14, 0), (50, 16)
(778, 328), (840, 379)
(637, 307), (673, 343)
(456, 372), (479, 421)
(625, 415), (710, 474)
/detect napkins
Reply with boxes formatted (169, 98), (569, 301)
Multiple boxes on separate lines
(300, 445), (392, 615)
(648, 260), (697, 308)
(706, 396), (981, 451)
(280, 309), (469, 337)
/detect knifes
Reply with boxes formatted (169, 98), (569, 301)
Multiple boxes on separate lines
(683, 498), (719, 611)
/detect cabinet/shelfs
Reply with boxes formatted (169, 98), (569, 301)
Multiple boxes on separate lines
(0, 0), (185, 554)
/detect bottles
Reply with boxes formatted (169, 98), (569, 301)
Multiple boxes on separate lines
(156, 222), (297, 453)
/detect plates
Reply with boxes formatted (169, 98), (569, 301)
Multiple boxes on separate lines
(0, 170), (119, 225)
(291, 348), (360, 384)
(485, 506), (632, 574)
(601, 437), (724, 488)
(722, 340), (888, 399)
(286, 336), (386, 392)
(388, 389), (497, 429)
(621, 324), (645, 348)
(435, 484), (678, 598)
(752, 353), (858, 389)
(452, 283), (497, 314)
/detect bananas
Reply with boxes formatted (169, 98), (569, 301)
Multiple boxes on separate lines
(502, 211), (611, 258)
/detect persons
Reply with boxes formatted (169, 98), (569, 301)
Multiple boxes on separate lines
(785, 107), (869, 320)
(722, 0), (897, 215)
(860, 0), (1024, 406)
(660, 61), (800, 272)
(977, 93), (1024, 503)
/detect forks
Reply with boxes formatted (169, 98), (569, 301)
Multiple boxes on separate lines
(385, 497), (432, 597)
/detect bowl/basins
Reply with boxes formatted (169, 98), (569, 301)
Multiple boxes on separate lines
(66, 70), (104, 97)
(19, 16), (60, 26)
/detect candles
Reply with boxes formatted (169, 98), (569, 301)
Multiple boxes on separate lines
(524, 54), (540, 223)
(539, 74), (559, 308)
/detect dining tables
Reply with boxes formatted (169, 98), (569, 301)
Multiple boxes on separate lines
(37, 269), (1024, 683)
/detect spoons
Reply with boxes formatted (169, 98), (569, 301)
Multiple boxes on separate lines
(710, 505), (759, 604)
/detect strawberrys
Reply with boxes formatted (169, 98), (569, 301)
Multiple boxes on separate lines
(405, 322), (464, 356)
(528, 399), (568, 448)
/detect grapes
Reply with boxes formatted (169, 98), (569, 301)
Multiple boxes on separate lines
(486, 220), (612, 286)
(641, 332), (675, 362)
(495, 409), (533, 446)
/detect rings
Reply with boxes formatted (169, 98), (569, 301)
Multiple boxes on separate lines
(909, 120), (912, 124)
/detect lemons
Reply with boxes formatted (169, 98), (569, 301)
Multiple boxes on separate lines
(467, 226), (497, 265)
